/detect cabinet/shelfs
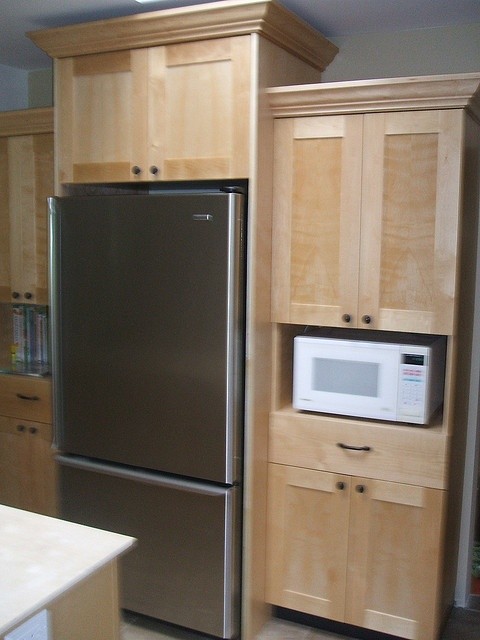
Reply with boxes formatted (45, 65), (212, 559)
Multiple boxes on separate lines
(65, 36), (259, 183)
(267, 408), (451, 639)
(273, 107), (462, 338)
(0, 134), (52, 519)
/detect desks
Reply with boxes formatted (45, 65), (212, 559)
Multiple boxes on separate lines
(1, 503), (142, 640)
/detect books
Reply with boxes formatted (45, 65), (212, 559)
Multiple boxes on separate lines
(11, 305), (50, 378)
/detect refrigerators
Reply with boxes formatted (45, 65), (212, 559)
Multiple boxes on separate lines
(45, 186), (240, 640)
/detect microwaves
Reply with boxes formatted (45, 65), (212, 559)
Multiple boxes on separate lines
(292, 327), (446, 426)
